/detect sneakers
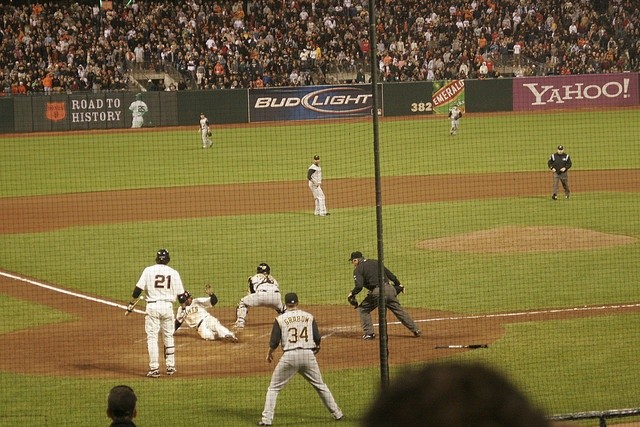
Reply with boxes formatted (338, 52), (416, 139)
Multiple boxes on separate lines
(414, 330), (421, 337)
(233, 320), (244, 330)
(167, 368), (177, 376)
(258, 420), (271, 426)
(362, 334), (375, 340)
(145, 369), (161, 378)
(225, 333), (238, 342)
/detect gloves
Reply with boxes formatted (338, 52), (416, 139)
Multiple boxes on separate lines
(127, 302), (134, 312)
(180, 308), (186, 319)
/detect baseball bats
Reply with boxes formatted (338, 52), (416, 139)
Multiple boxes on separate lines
(435, 343), (488, 349)
(124, 295), (144, 315)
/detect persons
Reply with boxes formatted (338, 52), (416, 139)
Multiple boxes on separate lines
(174, 286), (238, 344)
(307, 156), (329, 216)
(259, 293), (342, 427)
(106, 385), (137, 427)
(348, 252), (421, 339)
(232, 263), (285, 331)
(1, 0), (640, 98)
(126, 249), (186, 378)
(448, 105), (462, 135)
(128, 93), (149, 128)
(198, 112), (213, 148)
(548, 146), (572, 200)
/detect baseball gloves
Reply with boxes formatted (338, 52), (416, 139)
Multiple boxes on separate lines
(207, 130), (212, 137)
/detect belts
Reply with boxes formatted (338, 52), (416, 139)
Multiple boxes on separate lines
(148, 301), (156, 303)
(373, 282), (390, 288)
(197, 316), (207, 327)
(263, 290), (279, 293)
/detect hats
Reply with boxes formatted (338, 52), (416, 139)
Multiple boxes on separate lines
(314, 155), (319, 160)
(185, 291), (191, 300)
(348, 251), (363, 261)
(257, 263), (270, 274)
(108, 385), (137, 417)
(558, 145), (563, 149)
(155, 248), (170, 260)
(285, 293), (299, 307)
